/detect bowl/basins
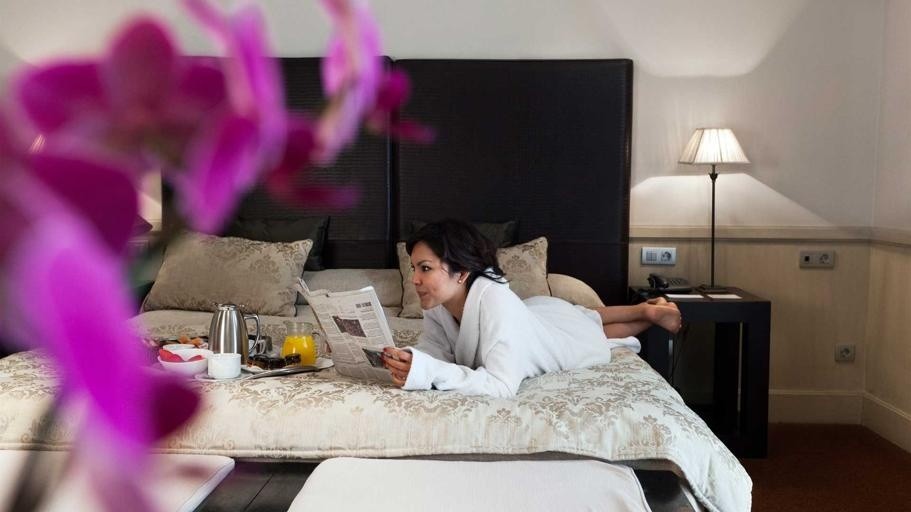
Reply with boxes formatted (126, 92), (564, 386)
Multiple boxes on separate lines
(156, 349), (214, 376)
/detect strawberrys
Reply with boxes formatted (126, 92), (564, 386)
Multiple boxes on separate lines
(158, 349), (203, 362)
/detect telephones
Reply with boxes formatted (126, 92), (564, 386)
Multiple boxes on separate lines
(647, 273), (692, 293)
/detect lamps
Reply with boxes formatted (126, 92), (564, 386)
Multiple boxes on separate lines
(679, 126), (749, 295)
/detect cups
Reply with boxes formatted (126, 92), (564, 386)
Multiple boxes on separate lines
(162, 343), (196, 351)
(207, 353), (242, 380)
(279, 320), (325, 368)
(247, 337), (266, 358)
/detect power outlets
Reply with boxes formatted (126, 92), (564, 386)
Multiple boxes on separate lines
(800, 250), (836, 268)
(834, 344), (856, 362)
(642, 247), (677, 267)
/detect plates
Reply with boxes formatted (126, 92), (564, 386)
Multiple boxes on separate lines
(241, 363), (269, 374)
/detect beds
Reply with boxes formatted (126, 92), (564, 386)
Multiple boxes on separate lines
(0, 244), (703, 512)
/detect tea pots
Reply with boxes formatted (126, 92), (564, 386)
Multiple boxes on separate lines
(207, 301), (261, 365)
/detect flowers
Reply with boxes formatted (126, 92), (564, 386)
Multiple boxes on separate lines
(1, 3), (437, 512)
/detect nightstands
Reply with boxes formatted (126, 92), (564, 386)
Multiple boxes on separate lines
(632, 285), (773, 469)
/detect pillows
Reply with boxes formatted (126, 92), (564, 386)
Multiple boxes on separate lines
(397, 236), (550, 319)
(141, 229), (317, 319)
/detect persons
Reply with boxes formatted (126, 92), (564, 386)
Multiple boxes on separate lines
(323, 218), (684, 398)
